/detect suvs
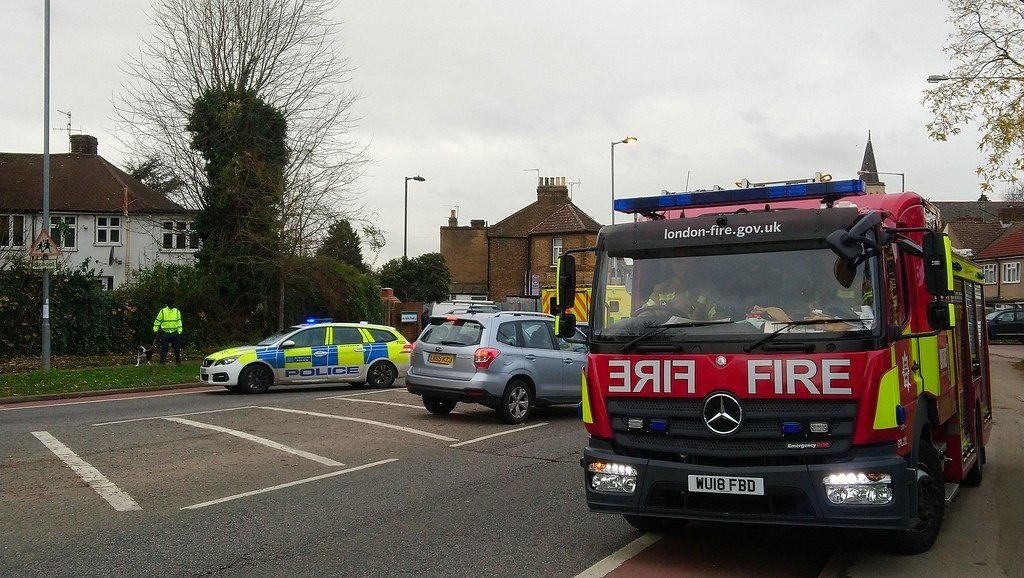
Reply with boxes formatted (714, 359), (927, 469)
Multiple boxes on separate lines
(406, 309), (589, 421)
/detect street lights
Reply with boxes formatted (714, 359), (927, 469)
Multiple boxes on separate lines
(403, 176), (425, 255)
(525, 168), (540, 184)
(857, 171), (904, 193)
(611, 137), (638, 224)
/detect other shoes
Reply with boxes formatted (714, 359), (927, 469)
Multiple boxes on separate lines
(174, 358), (181, 366)
(160, 362), (166, 365)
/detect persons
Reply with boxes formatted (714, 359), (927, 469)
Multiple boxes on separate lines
(153, 300), (182, 364)
(421, 308), (429, 340)
(815, 250), (873, 309)
(640, 257), (726, 321)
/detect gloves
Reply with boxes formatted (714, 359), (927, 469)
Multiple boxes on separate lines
(152, 332), (158, 339)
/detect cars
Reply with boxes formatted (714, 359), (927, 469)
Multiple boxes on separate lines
(199, 317), (412, 394)
(984, 309), (1024, 345)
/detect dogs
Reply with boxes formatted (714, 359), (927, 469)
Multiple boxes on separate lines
(135, 346), (156, 367)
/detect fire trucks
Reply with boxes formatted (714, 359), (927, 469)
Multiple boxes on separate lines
(549, 172), (994, 555)
